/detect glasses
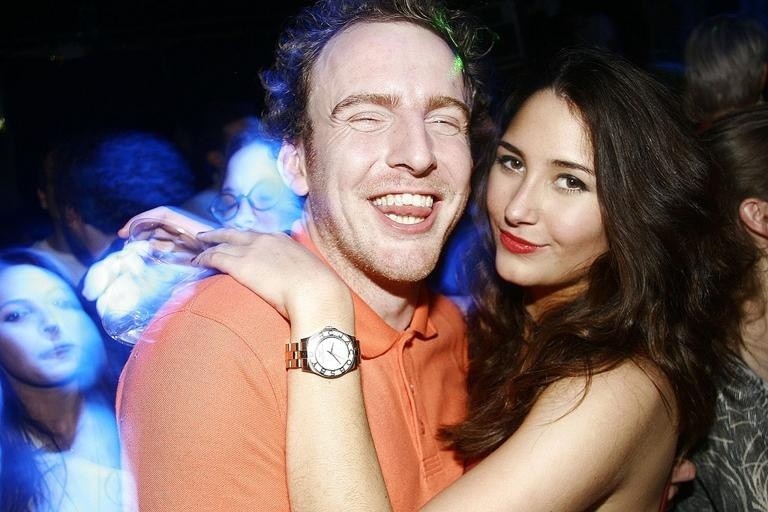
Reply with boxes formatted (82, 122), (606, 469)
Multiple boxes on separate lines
(209, 178), (283, 223)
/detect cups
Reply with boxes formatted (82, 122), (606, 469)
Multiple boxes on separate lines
(95, 216), (211, 347)
